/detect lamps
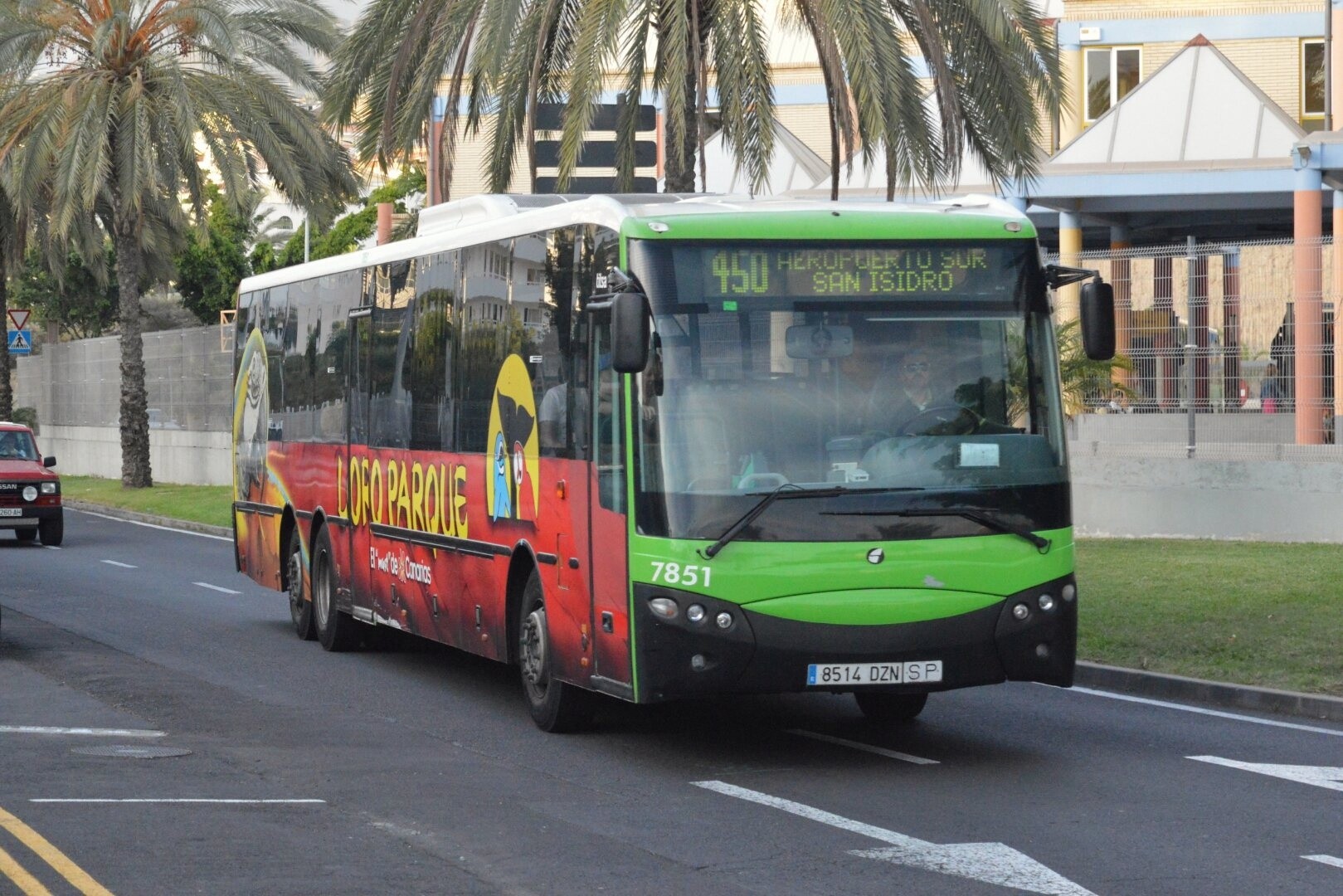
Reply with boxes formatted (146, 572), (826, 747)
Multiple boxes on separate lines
(1297, 147), (1312, 168)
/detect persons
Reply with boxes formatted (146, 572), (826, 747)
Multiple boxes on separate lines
(1224, 389), (1241, 412)
(1105, 390), (1163, 416)
(538, 343), (687, 451)
(1259, 357), (1282, 413)
(874, 349), (1035, 438)
(0, 434), (28, 459)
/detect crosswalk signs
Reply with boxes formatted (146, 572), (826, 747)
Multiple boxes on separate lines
(6, 330), (33, 354)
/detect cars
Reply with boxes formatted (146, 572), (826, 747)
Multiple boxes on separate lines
(0, 420), (65, 546)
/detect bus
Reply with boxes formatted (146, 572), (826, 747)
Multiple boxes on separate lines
(229, 189), (1118, 736)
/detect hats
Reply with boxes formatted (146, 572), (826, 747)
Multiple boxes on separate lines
(599, 352), (612, 371)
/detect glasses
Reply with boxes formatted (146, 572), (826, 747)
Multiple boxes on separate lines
(903, 362), (930, 372)
